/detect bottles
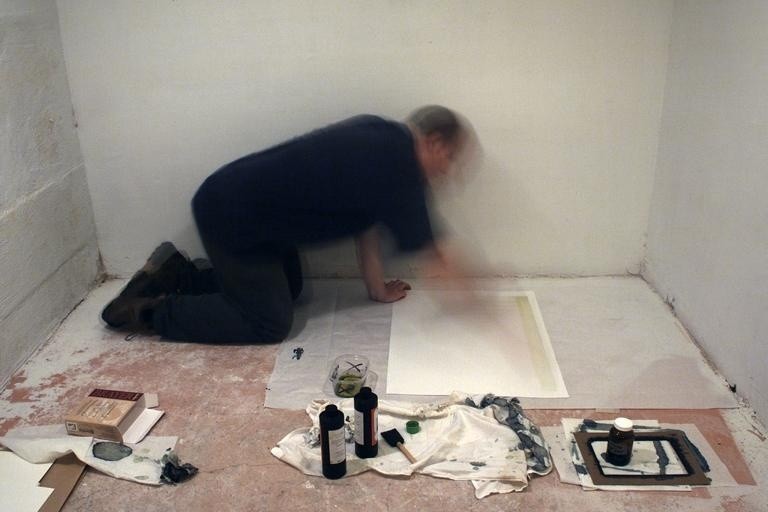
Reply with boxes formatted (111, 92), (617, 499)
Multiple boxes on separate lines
(607, 418), (635, 466)
(320, 404), (347, 480)
(353, 385), (379, 458)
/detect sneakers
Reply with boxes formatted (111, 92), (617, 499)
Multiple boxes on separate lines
(100, 241), (199, 342)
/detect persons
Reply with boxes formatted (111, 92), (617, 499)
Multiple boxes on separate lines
(101, 104), (468, 345)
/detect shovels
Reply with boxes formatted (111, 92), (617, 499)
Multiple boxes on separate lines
(380, 428), (418, 464)
